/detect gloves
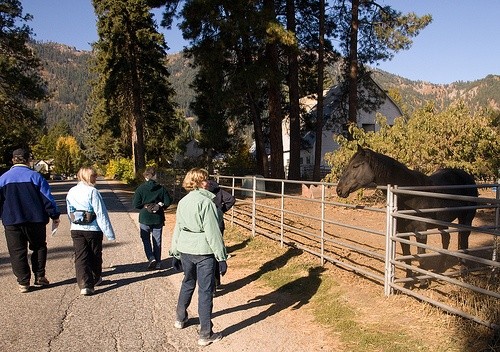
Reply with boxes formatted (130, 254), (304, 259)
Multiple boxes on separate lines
(218, 261), (227, 277)
(171, 257), (182, 272)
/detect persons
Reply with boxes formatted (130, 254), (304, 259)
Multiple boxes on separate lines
(65, 167), (116, 295)
(207, 180), (236, 297)
(0, 149), (61, 293)
(132, 166), (171, 269)
(169, 167), (228, 346)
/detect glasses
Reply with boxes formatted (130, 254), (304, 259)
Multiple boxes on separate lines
(90, 173), (98, 179)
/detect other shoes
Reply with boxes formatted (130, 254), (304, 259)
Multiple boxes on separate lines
(81, 288), (92, 295)
(148, 259), (162, 269)
(213, 293), (216, 297)
(18, 285), (30, 292)
(95, 276), (102, 286)
(34, 277), (49, 287)
(216, 285), (220, 289)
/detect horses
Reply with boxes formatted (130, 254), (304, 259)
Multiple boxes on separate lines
(337, 143), (479, 288)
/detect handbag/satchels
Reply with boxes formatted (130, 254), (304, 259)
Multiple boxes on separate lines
(145, 204), (161, 214)
(70, 210), (94, 224)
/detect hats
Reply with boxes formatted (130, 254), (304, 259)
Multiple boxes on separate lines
(13, 149), (30, 161)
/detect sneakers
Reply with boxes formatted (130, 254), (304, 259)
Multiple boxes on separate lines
(198, 332), (222, 345)
(174, 317), (188, 328)
